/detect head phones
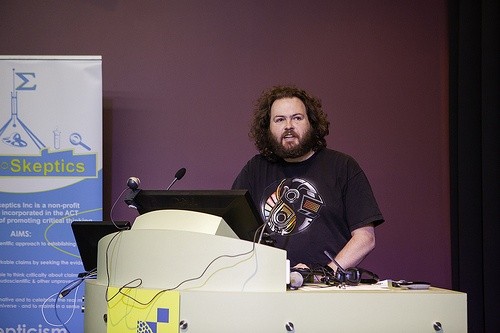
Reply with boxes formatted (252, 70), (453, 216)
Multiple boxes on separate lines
(335, 267), (379, 285)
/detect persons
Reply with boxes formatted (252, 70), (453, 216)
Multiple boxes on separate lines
(231, 85), (385, 283)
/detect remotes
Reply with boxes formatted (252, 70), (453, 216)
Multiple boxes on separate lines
(392, 282), (430, 287)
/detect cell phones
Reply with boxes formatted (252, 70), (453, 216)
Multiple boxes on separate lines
(398, 283), (431, 289)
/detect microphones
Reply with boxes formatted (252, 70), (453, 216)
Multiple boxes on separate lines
(167, 168), (186, 190)
(258, 179), (292, 244)
(324, 251), (346, 274)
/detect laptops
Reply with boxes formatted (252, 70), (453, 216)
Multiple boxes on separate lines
(71, 221), (130, 271)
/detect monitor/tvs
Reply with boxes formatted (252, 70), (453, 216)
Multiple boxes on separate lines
(134, 189), (268, 246)
